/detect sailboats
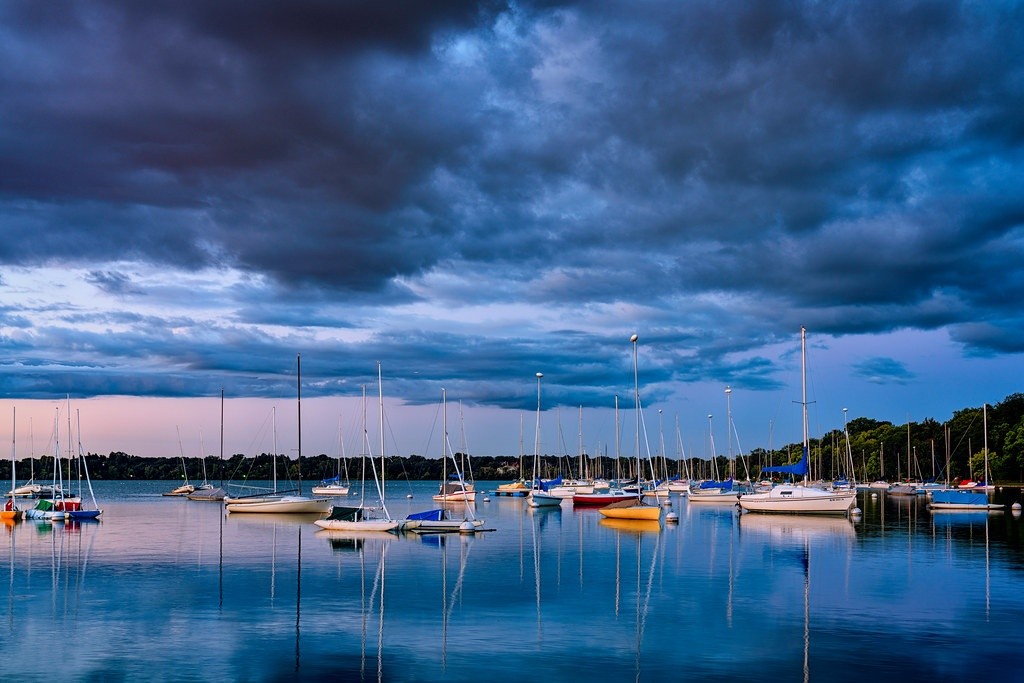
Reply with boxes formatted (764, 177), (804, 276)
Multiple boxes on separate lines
(0, 393), (105, 521)
(490, 325), (1006, 522)
(225, 353), (486, 534)
(162, 424), (228, 502)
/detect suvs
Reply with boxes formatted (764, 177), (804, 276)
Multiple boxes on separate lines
(449, 472), (460, 478)
